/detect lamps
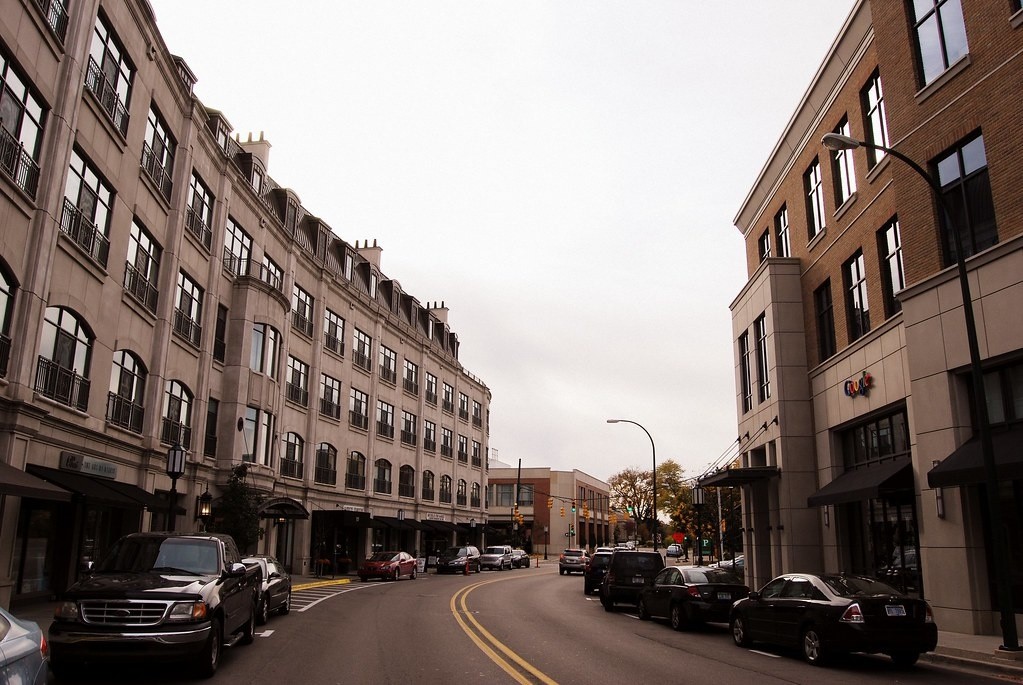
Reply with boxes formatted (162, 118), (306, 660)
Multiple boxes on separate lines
(746, 527), (753, 532)
(824, 505), (829, 527)
(739, 526), (745, 533)
(195, 480), (211, 526)
(932, 460), (944, 519)
(776, 520), (783, 530)
(766, 521), (772, 530)
(274, 511), (285, 528)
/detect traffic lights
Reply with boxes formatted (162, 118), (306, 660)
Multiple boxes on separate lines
(582, 505), (590, 520)
(548, 499), (553, 508)
(572, 502), (576, 512)
(684, 535), (688, 543)
(570, 524), (574, 533)
(608, 515), (617, 523)
(560, 507), (563, 517)
(627, 501), (633, 511)
(516, 509), (523, 525)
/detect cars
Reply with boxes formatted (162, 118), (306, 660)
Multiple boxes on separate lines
(0, 605), (48, 685)
(594, 541), (639, 554)
(237, 553), (292, 625)
(397, 509), (405, 552)
(356, 550), (418, 581)
(728, 572), (939, 667)
(634, 565), (751, 629)
(512, 549), (531, 568)
(666, 544), (684, 557)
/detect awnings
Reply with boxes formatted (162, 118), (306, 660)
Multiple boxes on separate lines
(806, 456), (912, 507)
(367, 515), (468, 534)
(28, 462), (187, 517)
(927, 418), (1023, 488)
(0, 460), (72, 501)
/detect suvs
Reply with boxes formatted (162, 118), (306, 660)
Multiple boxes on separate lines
(437, 545), (481, 573)
(583, 552), (613, 596)
(599, 550), (666, 611)
(559, 549), (591, 575)
(480, 545), (513, 571)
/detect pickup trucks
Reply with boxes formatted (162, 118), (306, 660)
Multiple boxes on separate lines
(48, 531), (264, 680)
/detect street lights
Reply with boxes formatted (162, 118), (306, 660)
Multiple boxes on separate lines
(470, 518), (477, 547)
(607, 419), (658, 551)
(165, 441), (186, 531)
(820, 132), (1023, 653)
